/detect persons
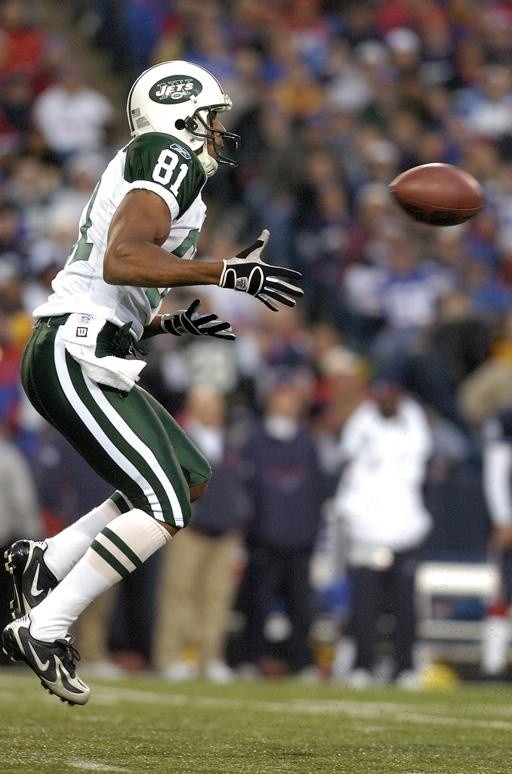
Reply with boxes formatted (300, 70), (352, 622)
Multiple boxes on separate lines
(0, 52), (309, 711)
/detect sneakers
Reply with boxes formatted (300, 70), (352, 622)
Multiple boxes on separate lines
(2, 539), (90, 705)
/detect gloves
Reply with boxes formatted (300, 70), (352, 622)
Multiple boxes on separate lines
(218, 229), (304, 312)
(161, 299), (235, 341)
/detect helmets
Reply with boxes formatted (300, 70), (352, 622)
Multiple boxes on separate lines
(126, 60), (241, 178)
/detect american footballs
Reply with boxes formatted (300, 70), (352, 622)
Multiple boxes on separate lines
(388, 163), (485, 224)
